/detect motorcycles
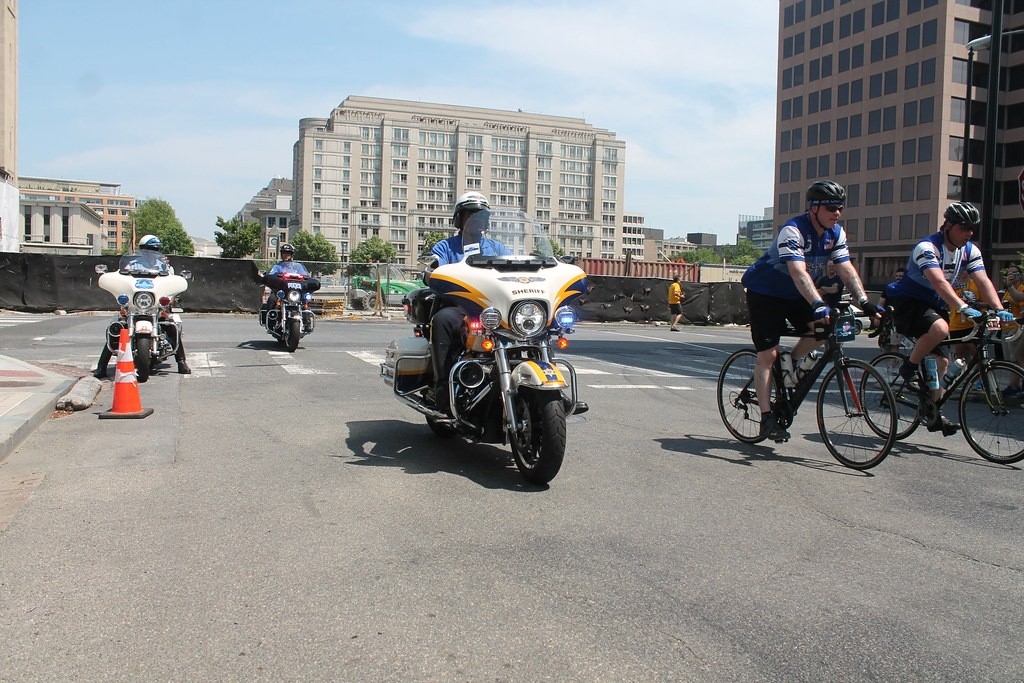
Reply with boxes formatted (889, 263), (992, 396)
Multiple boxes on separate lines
(95, 248), (192, 382)
(379, 207), (597, 486)
(257, 269), (319, 352)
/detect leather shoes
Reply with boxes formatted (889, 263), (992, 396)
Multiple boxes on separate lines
(434, 388), (451, 407)
(94, 368), (107, 377)
(560, 387), (589, 415)
(178, 361), (192, 374)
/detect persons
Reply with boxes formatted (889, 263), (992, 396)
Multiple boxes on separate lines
(815, 260), (844, 353)
(421, 191), (589, 415)
(668, 276), (685, 332)
(265, 244), (313, 333)
(948, 278), (982, 390)
(997, 266), (1024, 396)
(891, 201), (1015, 433)
(741, 180), (883, 440)
(93, 234), (191, 378)
(875, 268), (906, 373)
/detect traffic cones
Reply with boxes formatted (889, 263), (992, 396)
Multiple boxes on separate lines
(99, 329), (154, 418)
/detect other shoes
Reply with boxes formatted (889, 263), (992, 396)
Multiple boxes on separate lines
(670, 326), (680, 331)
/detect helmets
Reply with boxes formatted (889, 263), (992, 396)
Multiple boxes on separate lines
(942, 202), (981, 226)
(453, 192), (490, 232)
(806, 180), (847, 201)
(961, 291), (977, 304)
(138, 235), (163, 251)
(280, 244), (295, 262)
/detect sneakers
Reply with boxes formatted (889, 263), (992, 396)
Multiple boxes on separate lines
(928, 415), (961, 432)
(900, 357), (922, 393)
(759, 416), (790, 441)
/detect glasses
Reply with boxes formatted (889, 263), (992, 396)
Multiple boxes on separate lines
(826, 205), (843, 213)
(960, 225), (975, 231)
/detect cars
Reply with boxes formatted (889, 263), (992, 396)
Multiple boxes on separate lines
(785, 301), (871, 337)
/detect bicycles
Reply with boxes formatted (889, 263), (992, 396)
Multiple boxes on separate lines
(860, 309), (1024, 462)
(716, 294), (902, 470)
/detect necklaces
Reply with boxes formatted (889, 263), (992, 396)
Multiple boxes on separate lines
(960, 280), (972, 301)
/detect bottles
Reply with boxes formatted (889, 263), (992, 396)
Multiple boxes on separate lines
(925, 355), (939, 391)
(792, 350), (819, 387)
(939, 358), (967, 389)
(780, 347), (796, 387)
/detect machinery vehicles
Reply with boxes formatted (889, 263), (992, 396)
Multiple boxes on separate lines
(347, 263), (429, 311)
